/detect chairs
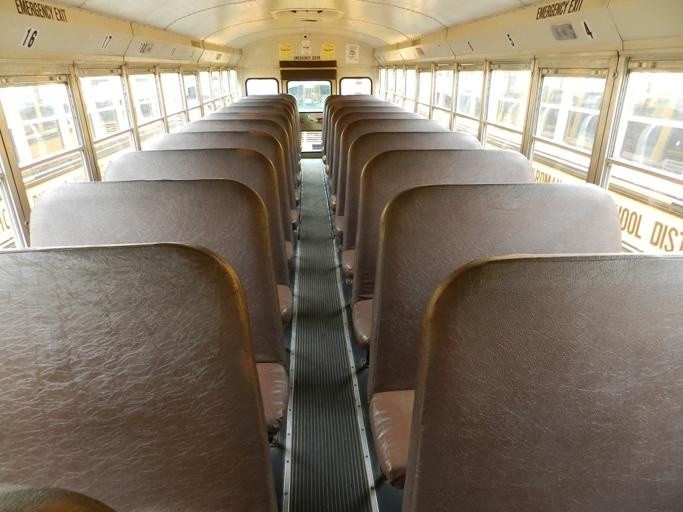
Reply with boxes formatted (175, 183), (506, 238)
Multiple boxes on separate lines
(323, 92), (532, 287)
(348, 143), (536, 349)
(1, 237), (285, 510)
(86, 96), (296, 330)
(20, 175), (294, 438)
(366, 178), (627, 489)
(395, 244), (683, 512)
(432, 137), (444, 141)
